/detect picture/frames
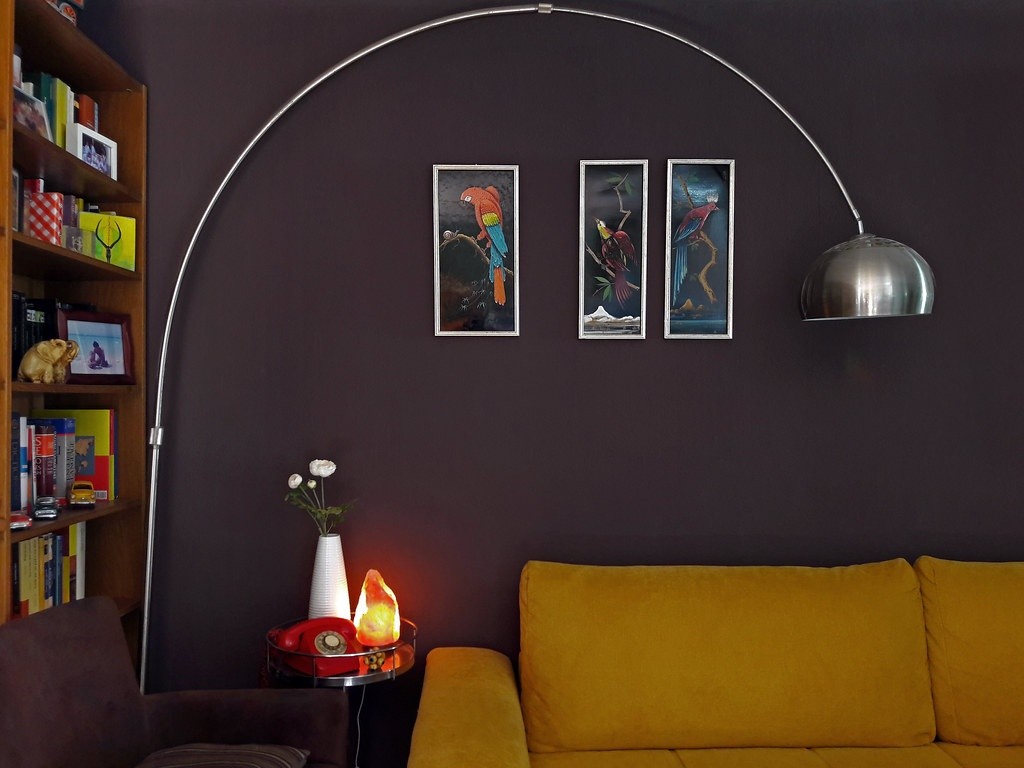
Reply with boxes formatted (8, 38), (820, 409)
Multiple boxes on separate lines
(55, 308), (137, 386)
(67, 122), (118, 182)
(430, 158), (735, 341)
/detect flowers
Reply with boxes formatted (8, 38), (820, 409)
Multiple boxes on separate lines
(284, 459), (342, 537)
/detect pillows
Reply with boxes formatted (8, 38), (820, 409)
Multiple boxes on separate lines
(135, 743), (310, 768)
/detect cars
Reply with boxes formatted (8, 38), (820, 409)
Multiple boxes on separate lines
(32, 496), (60, 519)
(69, 482), (97, 510)
(10, 510), (32, 531)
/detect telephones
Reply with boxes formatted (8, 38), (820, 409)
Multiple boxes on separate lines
(273, 614), (364, 680)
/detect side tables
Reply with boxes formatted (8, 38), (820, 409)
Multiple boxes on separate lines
(267, 613), (419, 768)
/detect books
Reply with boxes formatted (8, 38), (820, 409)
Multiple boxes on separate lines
(12, 170), (136, 272)
(11, 408), (120, 500)
(13, 54), (98, 150)
(12, 520), (86, 617)
(12, 290), (97, 385)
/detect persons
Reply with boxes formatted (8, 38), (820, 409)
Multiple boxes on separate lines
(87, 341), (108, 369)
(83, 141), (108, 173)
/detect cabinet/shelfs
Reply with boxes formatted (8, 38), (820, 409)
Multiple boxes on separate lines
(0, 0), (147, 668)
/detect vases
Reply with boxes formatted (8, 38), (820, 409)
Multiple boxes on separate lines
(308, 533), (352, 621)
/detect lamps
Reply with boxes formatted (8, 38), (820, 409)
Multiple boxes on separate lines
(137, 2), (938, 695)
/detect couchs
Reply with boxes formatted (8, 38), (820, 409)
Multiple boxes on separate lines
(404, 556), (1024, 768)
(0, 595), (350, 768)
